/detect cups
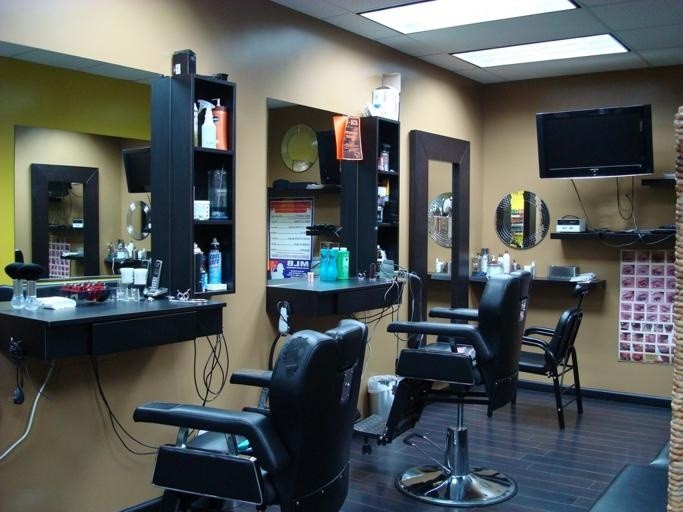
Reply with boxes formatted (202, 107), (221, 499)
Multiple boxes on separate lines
(206, 169), (230, 220)
(120, 287), (129, 301)
(436, 264), (444, 273)
(131, 289), (141, 301)
(379, 259), (395, 278)
(112, 287), (120, 299)
(193, 199), (210, 222)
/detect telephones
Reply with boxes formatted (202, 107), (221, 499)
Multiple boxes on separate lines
(144, 259), (168, 301)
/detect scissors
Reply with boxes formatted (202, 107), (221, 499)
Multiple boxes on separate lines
(357, 270), (366, 278)
(176, 289), (191, 300)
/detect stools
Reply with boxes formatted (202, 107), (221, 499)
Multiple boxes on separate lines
(648, 436), (669, 471)
(586, 464), (668, 512)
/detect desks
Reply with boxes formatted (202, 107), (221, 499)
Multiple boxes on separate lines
(266, 277), (405, 316)
(0, 296), (227, 360)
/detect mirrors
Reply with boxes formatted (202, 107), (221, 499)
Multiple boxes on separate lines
(126, 201), (151, 239)
(48, 181), (85, 278)
(266, 98), (344, 280)
(281, 123), (318, 173)
(428, 192), (452, 248)
(426, 160), (454, 274)
(0, 55), (152, 284)
(495, 191), (550, 250)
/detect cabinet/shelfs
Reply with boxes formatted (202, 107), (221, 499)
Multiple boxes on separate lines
(267, 184), (341, 236)
(357, 116), (400, 276)
(193, 74), (236, 295)
(469, 177), (676, 290)
(48, 224), (85, 260)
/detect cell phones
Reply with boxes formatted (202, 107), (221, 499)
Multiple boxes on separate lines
(152, 258), (163, 291)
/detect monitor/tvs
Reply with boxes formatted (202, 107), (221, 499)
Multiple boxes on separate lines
(317, 130), (343, 186)
(535, 104), (656, 179)
(49, 182), (71, 198)
(122, 147), (152, 194)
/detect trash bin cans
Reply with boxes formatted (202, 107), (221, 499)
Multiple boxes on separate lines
(366, 374), (401, 421)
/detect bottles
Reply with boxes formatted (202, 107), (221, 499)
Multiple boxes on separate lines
(208, 237), (223, 285)
(497, 253), (503, 264)
(531, 261), (536, 279)
(137, 251), (143, 259)
(143, 247), (147, 259)
(319, 246), (329, 281)
(475, 253), (482, 260)
(329, 246), (340, 282)
(193, 242), (204, 294)
(487, 262), (503, 275)
(503, 250), (510, 273)
(472, 258), (481, 275)
(193, 102), (198, 148)
(200, 256), (208, 290)
(128, 240), (134, 258)
(481, 247), (488, 273)
(133, 260), (150, 286)
(381, 143), (391, 171)
(119, 261), (133, 284)
(337, 247), (351, 280)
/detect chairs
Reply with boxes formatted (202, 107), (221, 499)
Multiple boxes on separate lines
(511, 273), (596, 430)
(133, 320), (367, 511)
(354, 272), (530, 505)
